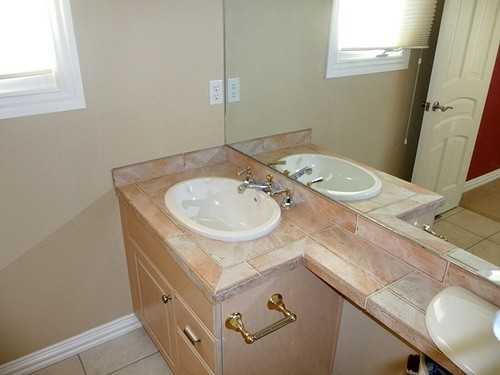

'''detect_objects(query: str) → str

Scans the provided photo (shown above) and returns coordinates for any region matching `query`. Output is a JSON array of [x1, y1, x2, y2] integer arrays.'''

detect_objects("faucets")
[[238, 174, 275, 196], [284, 166, 312, 181]]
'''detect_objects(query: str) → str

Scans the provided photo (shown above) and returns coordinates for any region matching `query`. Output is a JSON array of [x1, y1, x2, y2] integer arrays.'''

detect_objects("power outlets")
[[227, 78, 240, 102], [209, 80, 222, 106]]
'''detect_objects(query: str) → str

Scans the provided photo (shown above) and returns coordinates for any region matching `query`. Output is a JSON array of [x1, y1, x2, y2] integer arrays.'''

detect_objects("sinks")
[[276, 154, 382, 202], [164, 177, 281, 242]]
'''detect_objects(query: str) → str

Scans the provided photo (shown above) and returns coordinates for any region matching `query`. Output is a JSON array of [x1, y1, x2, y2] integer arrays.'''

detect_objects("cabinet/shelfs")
[[120, 202, 344, 375]]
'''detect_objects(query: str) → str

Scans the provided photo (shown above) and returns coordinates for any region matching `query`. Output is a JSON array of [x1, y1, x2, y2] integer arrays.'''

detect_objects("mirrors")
[[222, 0, 500, 285]]
